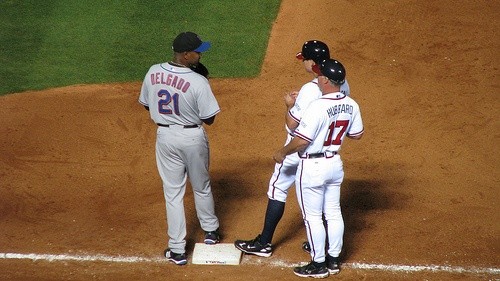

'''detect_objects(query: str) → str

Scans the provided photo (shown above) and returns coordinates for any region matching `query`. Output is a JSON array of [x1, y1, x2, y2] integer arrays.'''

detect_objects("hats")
[[172, 32, 211, 52]]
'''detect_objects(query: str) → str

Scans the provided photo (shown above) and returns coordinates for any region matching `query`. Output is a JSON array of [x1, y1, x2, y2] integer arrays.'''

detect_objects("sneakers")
[[302, 242, 326, 257], [293, 261, 328, 278], [325, 254, 339, 274], [204, 227, 221, 243], [234, 234, 272, 257], [164, 248, 187, 264]]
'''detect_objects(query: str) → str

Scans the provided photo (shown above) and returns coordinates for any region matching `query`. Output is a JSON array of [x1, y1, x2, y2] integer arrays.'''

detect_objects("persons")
[[139, 31, 223, 266], [235, 39, 350, 258], [273, 59, 364, 279]]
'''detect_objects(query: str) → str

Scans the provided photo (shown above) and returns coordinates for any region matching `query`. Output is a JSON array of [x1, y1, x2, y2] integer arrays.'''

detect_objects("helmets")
[[296, 40, 329, 64], [312, 59, 345, 84]]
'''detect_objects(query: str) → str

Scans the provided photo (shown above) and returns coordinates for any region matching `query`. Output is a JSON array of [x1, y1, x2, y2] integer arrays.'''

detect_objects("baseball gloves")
[[188, 62, 208, 77]]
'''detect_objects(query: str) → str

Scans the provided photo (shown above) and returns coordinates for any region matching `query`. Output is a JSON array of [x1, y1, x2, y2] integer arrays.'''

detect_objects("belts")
[[157, 123, 198, 128], [300, 152, 337, 158]]
